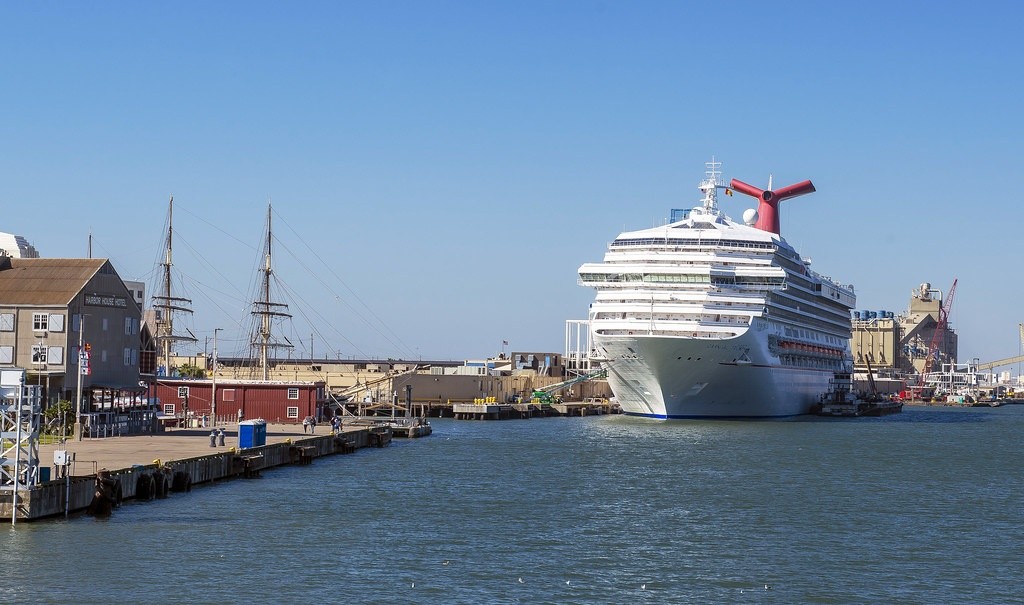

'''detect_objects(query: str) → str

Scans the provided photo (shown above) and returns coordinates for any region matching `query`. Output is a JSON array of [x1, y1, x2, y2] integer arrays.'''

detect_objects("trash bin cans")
[[238, 419, 266, 448]]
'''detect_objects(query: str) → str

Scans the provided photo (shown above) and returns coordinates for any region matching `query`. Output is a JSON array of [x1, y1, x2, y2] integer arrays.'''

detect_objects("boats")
[[810, 351, 904, 418]]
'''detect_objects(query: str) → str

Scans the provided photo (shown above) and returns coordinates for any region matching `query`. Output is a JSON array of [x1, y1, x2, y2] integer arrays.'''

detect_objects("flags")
[[725, 187, 733, 197], [503, 339, 508, 346], [79, 343, 92, 376]]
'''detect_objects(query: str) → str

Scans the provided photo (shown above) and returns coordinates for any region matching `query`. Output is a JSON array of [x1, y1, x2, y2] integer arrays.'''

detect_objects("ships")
[[578, 149, 856, 416]]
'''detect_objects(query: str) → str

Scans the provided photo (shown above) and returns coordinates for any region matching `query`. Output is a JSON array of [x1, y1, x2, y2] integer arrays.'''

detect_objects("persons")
[[302, 416, 308, 433], [201, 413, 207, 428], [330, 416, 343, 434], [308, 415, 317, 434]]
